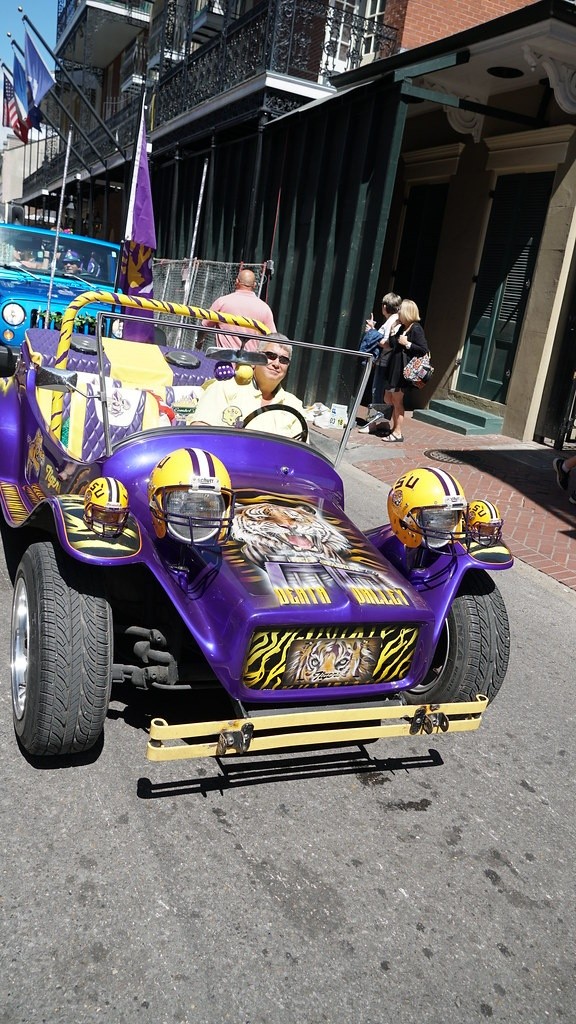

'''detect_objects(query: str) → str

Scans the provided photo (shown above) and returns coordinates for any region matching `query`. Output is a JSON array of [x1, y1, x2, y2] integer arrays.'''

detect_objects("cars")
[[0, 286, 513, 772]]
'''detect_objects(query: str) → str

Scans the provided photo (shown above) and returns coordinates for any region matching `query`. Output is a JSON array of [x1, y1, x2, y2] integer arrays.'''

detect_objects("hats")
[[61, 249, 83, 262]]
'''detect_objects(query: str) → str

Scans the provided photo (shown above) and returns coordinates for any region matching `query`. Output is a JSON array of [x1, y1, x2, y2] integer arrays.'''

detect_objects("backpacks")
[[400, 321, 436, 393]]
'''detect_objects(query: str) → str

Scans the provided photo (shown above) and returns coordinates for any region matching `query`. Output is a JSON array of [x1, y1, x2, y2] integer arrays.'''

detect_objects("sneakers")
[[552, 458, 572, 491], [569, 490, 576, 504], [357, 421, 377, 433], [376, 421, 391, 430]]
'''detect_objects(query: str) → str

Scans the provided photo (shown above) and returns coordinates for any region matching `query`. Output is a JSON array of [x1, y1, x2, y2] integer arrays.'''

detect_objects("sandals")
[[375, 430, 393, 436], [381, 433, 404, 442]]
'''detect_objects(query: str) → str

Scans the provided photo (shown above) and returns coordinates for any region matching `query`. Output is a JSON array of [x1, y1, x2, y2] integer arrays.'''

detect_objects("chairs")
[[40, 366, 169, 462], [157, 384, 204, 430]]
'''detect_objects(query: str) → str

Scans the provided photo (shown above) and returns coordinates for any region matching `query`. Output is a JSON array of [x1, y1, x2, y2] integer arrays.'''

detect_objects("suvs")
[[0, 223, 124, 374]]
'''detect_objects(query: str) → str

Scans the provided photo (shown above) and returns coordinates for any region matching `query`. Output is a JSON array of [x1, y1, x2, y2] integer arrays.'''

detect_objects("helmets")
[[146, 447, 234, 541], [387, 467, 469, 558], [83, 477, 129, 538], [467, 499, 502, 547]]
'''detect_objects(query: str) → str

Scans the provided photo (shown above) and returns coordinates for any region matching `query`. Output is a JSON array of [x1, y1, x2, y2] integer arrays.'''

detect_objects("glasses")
[[261, 350, 291, 364], [62, 261, 76, 265]]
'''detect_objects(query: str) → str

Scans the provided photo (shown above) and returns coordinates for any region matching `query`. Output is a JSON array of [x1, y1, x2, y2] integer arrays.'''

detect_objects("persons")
[[359, 292, 430, 442], [201, 269, 277, 354], [185, 331, 311, 453], [60, 253, 81, 274]]
